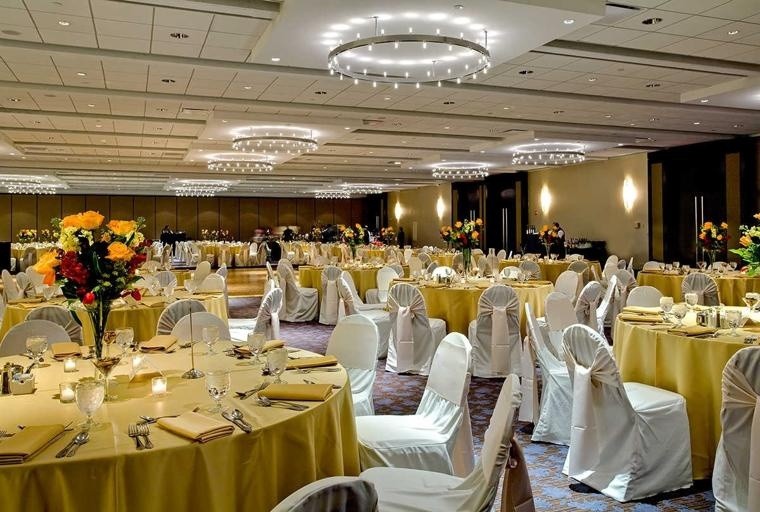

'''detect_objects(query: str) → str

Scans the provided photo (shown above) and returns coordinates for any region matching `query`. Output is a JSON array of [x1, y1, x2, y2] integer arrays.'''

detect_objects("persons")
[[282, 224, 294, 243], [550, 221, 566, 258], [361, 229, 370, 245], [396, 227, 405, 249], [161, 224, 173, 235], [320, 224, 337, 243]]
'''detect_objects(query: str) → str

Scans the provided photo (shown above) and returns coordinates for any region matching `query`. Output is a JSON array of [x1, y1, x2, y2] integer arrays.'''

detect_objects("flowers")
[[538, 224, 559, 250], [697, 213, 760, 276], [16, 228, 37, 243], [338, 223, 395, 250], [30, 210, 154, 357], [440, 218, 484, 274]]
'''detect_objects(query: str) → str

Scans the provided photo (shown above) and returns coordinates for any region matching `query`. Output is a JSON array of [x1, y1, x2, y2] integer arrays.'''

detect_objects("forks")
[[250, 394, 309, 412], [241, 382, 270, 400], [128, 424, 144, 451], [67, 434, 90, 458], [139, 423, 154, 450]]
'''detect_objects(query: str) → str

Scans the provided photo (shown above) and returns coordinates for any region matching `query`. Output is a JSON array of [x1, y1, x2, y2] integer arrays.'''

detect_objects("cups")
[[151, 377, 167, 397], [63, 357, 76, 372], [59, 382, 75, 402]]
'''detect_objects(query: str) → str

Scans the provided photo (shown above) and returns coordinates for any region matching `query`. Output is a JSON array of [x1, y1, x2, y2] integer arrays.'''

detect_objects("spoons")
[[304, 379, 341, 389], [140, 406, 201, 422], [232, 408, 252, 428], [236, 389, 257, 396]]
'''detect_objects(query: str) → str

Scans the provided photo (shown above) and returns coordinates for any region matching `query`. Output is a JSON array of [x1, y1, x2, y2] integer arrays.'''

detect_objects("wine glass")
[[410, 266, 536, 289], [660, 290, 760, 335], [184, 279, 197, 296], [25, 326, 294, 430], [44, 288, 53, 301], [279, 239, 482, 256], [658, 260, 739, 278], [312, 254, 401, 270], [163, 286, 174, 304], [508, 251, 587, 264]]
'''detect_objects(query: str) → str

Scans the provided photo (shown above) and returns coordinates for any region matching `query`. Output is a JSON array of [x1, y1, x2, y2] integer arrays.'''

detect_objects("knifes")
[[56, 429, 88, 459], [220, 412, 251, 434]]
